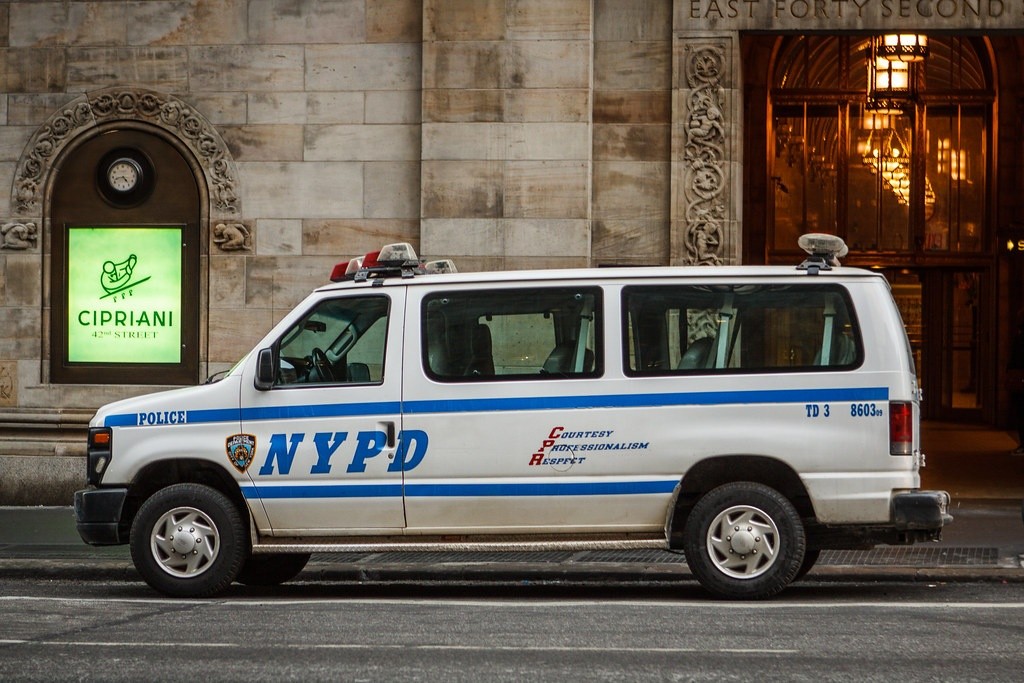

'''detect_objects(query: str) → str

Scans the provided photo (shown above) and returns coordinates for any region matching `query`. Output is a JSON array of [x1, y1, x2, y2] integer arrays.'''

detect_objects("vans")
[[72, 244, 956, 602]]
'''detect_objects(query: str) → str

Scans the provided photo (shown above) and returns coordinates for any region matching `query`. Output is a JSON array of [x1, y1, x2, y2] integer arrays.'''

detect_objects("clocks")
[[106, 158, 144, 194]]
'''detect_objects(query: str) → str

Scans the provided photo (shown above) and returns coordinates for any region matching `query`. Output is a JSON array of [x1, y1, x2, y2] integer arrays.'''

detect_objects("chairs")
[[679, 336, 714, 373], [537, 341, 596, 373], [425, 312, 453, 378], [464, 322, 496, 379], [813, 332, 856, 365]]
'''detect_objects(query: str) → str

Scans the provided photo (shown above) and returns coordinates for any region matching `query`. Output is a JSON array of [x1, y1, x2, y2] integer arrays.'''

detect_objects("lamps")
[[866, 57, 914, 117], [861, 112, 969, 207], [870, 46, 916, 98], [879, 32, 930, 63], [777, 124, 837, 189]]
